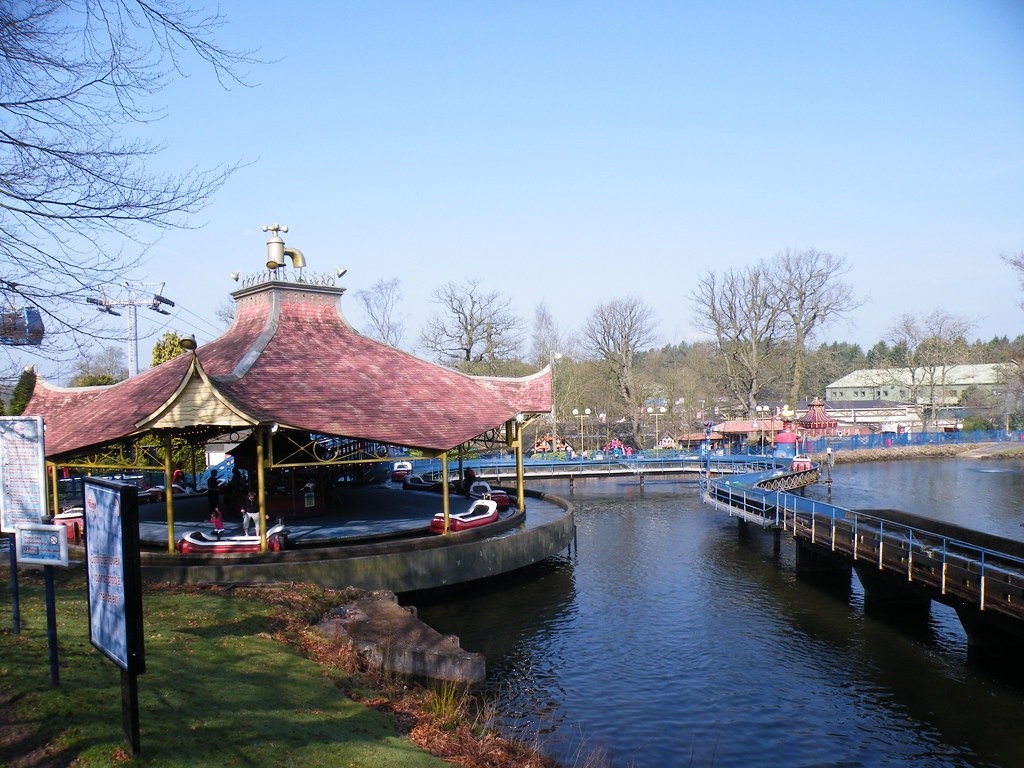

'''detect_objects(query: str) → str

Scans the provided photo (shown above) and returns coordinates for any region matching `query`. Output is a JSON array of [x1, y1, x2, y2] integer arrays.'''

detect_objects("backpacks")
[[464, 470, 475, 478]]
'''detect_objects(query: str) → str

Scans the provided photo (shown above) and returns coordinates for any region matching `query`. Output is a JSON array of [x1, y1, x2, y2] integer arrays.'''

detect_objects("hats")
[[211, 469, 218, 474]]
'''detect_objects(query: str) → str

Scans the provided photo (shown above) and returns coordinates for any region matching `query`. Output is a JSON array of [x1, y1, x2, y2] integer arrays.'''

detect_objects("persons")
[[208, 507, 225, 541], [241, 492, 260, 537], [208, 470, 223, 515], [222, 467, 241, 519], [463, 468, 476, 500]]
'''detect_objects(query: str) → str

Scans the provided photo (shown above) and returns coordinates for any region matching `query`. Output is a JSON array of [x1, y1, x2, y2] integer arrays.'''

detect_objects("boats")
[[392, 461, 413, 481], [469, 481, 509, 508], [428, 499, 499, 534], [138, 479, 227, 505], [402, 473, 455, 492], [53, 507, 84, 538], [176, 523, 287, 553]]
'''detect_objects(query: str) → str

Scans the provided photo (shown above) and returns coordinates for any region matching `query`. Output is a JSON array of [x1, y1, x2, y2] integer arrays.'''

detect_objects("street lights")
[[572, 406, 592, 463], [756, 405, 770, 455], [644, 406, 666, 457]]
